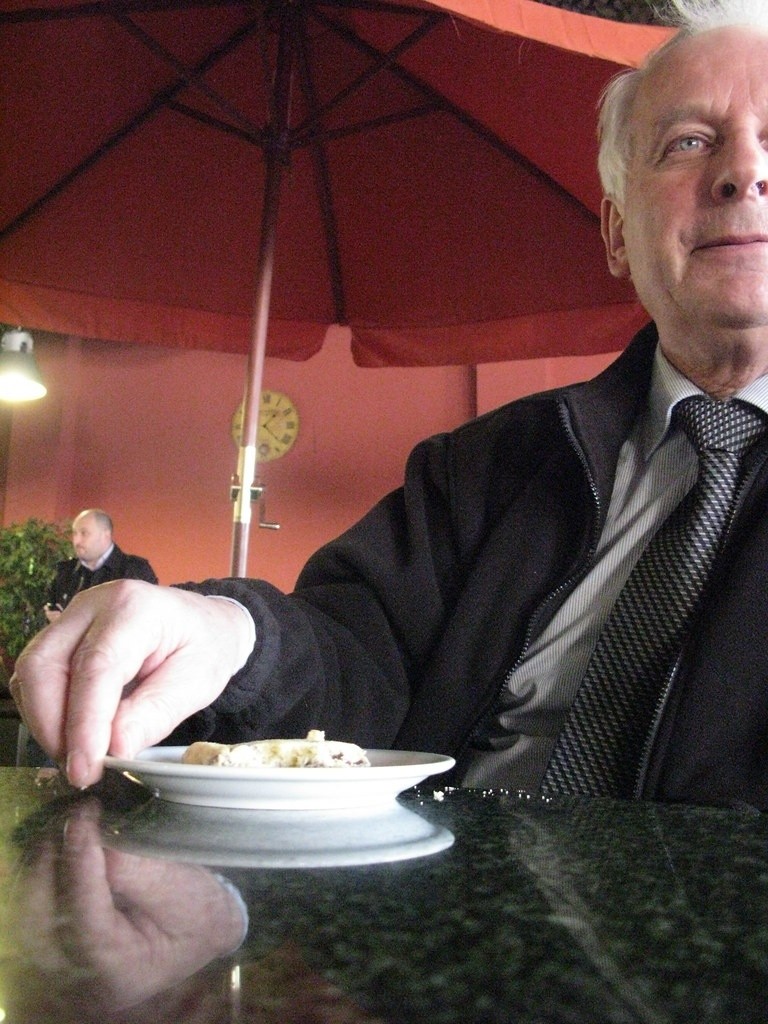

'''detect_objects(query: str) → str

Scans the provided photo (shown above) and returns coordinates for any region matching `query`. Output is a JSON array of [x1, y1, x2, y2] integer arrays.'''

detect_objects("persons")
[[10, 768, 768, 1024], [8, 23, 768, 797], [44, 509, 159, 622]]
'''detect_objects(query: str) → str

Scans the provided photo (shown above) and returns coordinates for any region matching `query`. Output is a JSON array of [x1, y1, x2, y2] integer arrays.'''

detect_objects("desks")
[[0, 763, 768, 1023]]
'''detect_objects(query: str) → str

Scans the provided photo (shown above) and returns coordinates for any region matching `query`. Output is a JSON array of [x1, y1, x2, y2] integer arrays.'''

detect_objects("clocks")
[[231, 387, 297, 462]]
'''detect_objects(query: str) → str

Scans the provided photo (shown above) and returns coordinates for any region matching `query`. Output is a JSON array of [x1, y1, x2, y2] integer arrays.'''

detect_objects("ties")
[[540, 400, 768, 800]]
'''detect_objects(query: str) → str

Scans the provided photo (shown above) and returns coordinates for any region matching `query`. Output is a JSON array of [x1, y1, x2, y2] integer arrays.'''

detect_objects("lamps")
[[0, 325, 46, 406]]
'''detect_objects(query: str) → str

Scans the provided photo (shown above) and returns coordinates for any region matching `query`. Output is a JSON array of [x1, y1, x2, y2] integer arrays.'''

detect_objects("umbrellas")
[[1, 1, 682, 590]]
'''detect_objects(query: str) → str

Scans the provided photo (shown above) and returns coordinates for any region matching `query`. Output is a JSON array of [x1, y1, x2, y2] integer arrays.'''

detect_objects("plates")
[[103, 746, 456, 809], [101, 798, 455, 870]]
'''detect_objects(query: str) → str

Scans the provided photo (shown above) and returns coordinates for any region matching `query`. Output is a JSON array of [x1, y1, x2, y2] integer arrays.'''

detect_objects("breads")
[[182, 730, 372, 769]]
